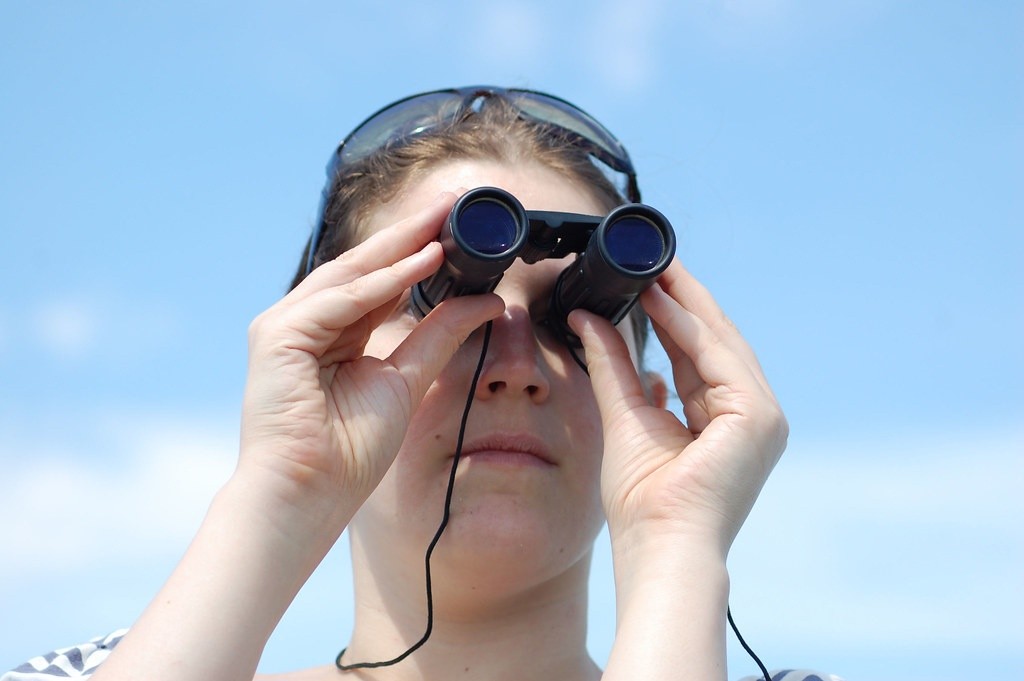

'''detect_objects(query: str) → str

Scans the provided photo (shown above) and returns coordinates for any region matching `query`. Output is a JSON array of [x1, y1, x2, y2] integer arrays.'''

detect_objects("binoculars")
[[410, 187, 675, 344]]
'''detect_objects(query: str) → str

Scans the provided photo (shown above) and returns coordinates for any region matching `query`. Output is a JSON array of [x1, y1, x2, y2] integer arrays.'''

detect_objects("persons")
[[0, 85, 828, 681]]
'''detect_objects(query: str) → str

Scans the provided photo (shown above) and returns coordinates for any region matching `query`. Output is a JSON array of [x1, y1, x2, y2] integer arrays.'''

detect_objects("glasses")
[[303, 85, 645, 285]]
